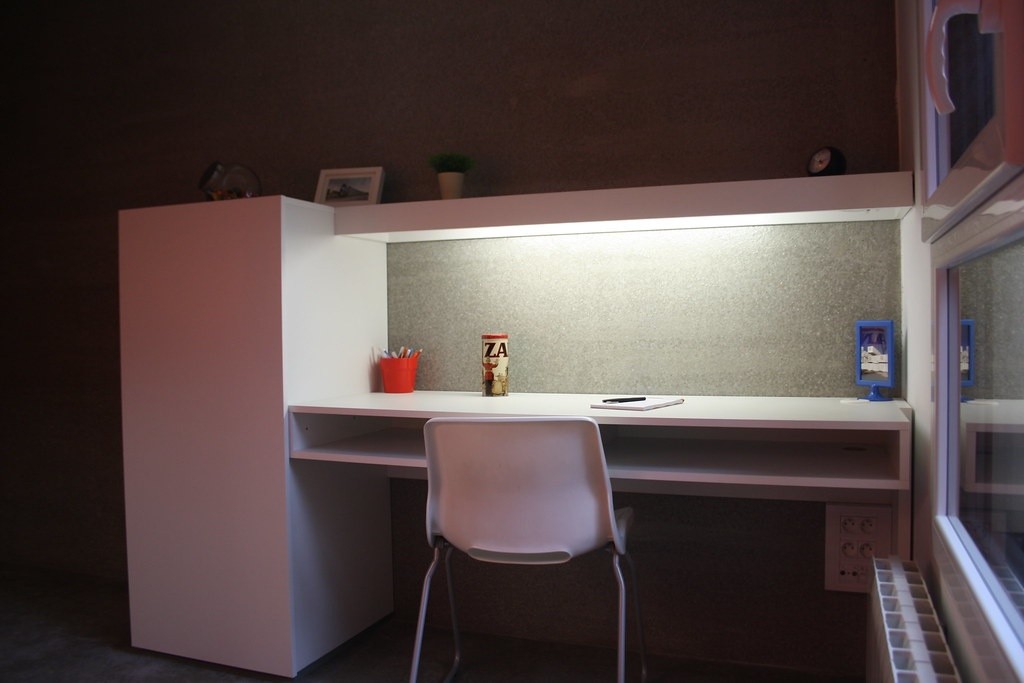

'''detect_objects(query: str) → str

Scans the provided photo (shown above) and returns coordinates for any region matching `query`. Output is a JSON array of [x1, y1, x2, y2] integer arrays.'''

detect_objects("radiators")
[[864, 556, 964, 683]]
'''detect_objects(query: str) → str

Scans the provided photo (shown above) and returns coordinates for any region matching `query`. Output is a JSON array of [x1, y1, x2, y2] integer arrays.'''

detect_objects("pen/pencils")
[[384, 346, 423, 358], [602, 397, 646, 403]]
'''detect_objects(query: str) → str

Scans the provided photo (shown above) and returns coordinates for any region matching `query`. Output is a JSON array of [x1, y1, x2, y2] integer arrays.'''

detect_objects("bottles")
[[199, 162, 262, 202], [482, 334, 509, 396]]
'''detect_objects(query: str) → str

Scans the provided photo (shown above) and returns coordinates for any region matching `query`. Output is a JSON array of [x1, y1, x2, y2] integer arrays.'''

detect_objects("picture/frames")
[[854, 321, 895, 387], [961, 319, 975, 388], [315, 165, 386, 206]]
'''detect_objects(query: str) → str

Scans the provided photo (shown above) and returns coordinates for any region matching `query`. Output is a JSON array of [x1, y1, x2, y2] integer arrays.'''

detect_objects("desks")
[[287, 392, 915, 560], [958, 400, 1024, 494]]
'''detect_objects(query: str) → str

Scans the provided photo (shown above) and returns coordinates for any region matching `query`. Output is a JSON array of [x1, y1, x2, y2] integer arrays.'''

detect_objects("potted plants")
[[431, 153, 468, 197]]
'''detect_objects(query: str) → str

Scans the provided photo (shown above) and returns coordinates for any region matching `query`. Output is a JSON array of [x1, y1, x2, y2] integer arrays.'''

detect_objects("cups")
[[380, 357, 418, 393]]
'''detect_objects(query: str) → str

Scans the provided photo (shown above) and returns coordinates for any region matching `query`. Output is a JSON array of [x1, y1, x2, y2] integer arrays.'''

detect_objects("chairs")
[[406, 412, 627, 682]]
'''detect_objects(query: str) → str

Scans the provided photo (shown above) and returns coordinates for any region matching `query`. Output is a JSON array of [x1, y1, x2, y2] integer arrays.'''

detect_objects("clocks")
[[805, 147, 845, 177]]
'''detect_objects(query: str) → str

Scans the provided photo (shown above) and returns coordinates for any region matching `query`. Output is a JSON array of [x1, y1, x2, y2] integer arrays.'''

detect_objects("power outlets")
[[824, 502, 892, 593]]
